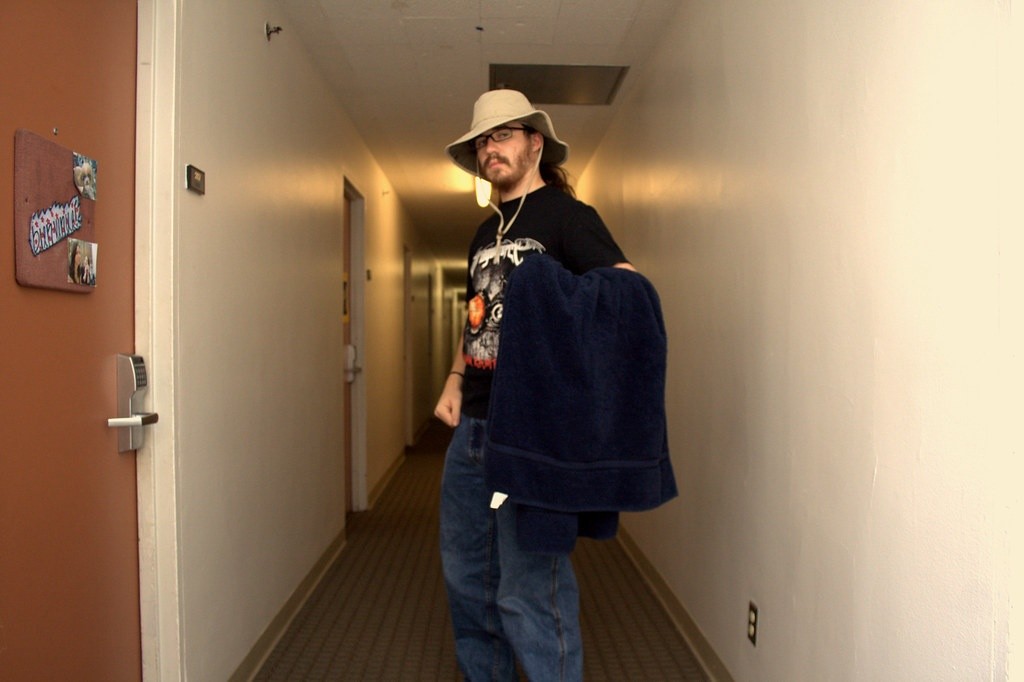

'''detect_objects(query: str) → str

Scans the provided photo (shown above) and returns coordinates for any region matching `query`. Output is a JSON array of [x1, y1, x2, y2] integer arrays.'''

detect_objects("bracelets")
[[450, 370, 465, 378]]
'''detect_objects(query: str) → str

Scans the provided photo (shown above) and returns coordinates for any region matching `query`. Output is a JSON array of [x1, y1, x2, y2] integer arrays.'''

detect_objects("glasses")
[[475, 125, 527, 149]]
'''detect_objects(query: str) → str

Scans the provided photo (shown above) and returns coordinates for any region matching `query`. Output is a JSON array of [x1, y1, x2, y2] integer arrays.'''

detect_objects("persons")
[[434, 88, 637, 682]]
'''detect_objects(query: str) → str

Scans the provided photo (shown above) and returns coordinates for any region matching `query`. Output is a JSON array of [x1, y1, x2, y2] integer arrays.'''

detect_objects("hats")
[[443, 89, 569, 182]]
